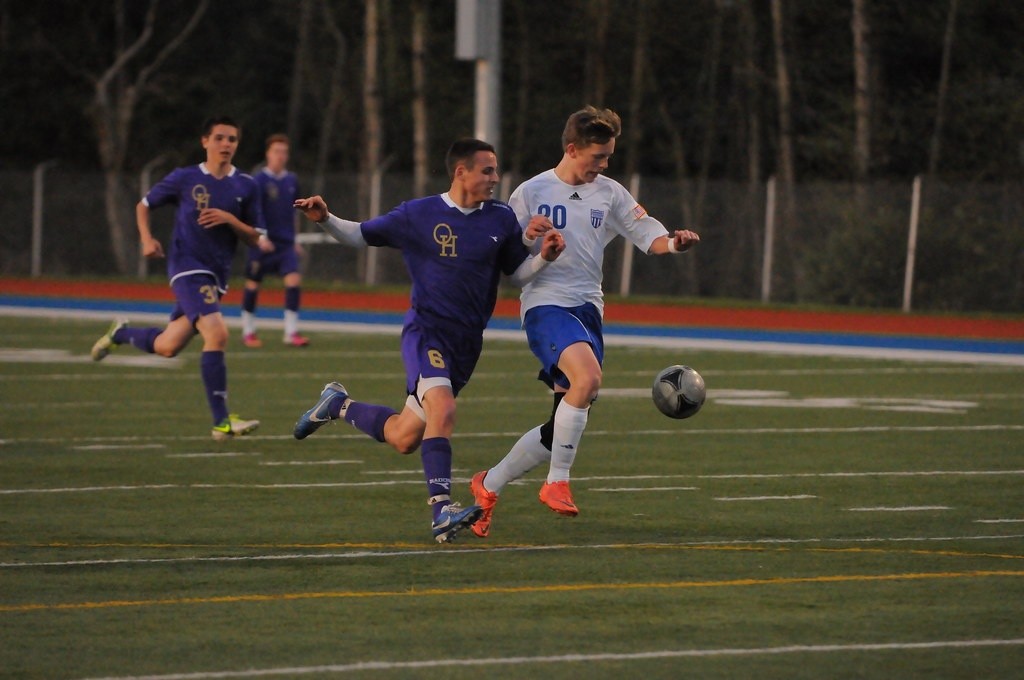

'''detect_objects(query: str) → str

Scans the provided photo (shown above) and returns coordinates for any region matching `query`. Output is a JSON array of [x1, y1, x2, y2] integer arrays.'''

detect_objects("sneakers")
[[539, 480, 579, 518], [92, 315, 129, 363], [244, 333, 262, 347], [282, 333, 310, 346], [431, 502, 483, 543], [293, 381, 348, 440], [469, 471, 498, 538], [212, 412, 260, 443]]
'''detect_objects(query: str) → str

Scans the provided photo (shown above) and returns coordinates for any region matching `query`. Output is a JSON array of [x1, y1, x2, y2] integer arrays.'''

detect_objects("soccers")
[[652, 363, 708, 420]]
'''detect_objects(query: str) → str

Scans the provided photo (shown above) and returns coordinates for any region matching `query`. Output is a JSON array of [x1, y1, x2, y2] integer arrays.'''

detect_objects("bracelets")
[[522, 226, 537, 246], [668, 238, 688, 255]]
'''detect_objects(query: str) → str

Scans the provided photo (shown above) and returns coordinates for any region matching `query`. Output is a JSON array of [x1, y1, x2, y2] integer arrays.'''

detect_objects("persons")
[[469, 105, 700, 537], [240, 134, 313, 349], [294, 136, 567, 545], [90, 114, 266, 439]]
